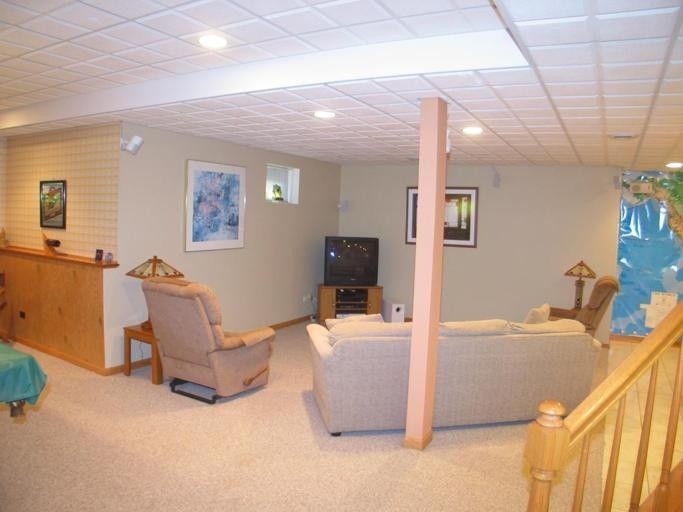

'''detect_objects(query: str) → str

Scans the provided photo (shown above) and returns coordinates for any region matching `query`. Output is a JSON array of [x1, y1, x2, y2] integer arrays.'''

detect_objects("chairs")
[[573, 274, 619, 337], [142, 277, 277, 407]]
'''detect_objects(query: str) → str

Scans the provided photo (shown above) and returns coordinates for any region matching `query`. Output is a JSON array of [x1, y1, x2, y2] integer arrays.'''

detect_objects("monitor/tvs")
[[324, 236, 380, 286]]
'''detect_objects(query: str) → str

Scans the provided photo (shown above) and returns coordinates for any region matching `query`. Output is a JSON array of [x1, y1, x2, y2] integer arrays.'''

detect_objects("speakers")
[[383, 297, 405, 323]]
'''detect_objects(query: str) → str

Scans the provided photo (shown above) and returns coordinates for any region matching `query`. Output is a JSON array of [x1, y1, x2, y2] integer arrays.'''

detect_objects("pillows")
[[525, 302, 550, 323], [323, 313, 384, 331]]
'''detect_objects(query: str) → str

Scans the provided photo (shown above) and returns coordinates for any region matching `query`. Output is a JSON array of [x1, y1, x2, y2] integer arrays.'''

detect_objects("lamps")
[[562, 261, 596, 310], [126, 255, 184, 331], [119, 132, 146, 157]]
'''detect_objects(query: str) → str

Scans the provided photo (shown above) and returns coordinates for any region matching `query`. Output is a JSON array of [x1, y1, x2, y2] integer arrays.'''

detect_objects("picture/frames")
[[183, 158, 247, 253], [405, 185, 478, 248], [39, 180, 67, 231]]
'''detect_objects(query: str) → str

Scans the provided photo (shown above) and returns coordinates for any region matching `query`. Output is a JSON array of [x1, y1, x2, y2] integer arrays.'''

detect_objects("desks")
[[123, 320, 165, 384], [549, 307, 577, 319], [0, 341, 50, 419]]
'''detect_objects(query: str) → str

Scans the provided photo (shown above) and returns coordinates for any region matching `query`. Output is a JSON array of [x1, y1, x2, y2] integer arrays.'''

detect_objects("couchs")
[[306, 319, 599, 438]]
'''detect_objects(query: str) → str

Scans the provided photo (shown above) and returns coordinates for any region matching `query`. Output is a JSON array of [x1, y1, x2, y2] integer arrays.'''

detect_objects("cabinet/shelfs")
[[317, 284, 384, 324]]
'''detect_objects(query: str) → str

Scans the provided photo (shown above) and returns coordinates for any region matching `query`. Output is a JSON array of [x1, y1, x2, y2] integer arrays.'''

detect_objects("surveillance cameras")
[[125, 134, 146, 156]]
